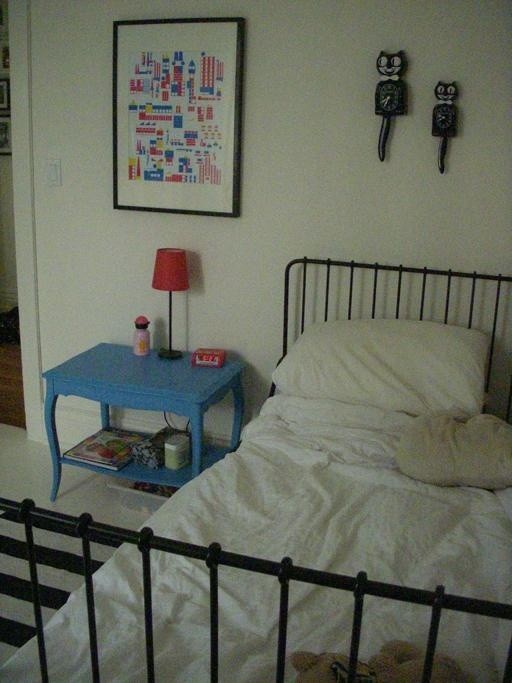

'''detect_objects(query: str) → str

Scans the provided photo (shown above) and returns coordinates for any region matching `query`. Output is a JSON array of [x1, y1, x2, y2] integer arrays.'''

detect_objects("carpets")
[[0, 508, 124, 663]]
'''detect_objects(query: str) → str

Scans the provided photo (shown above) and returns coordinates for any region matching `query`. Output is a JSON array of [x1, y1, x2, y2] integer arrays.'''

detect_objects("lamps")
[[151, 246, 190, 360]]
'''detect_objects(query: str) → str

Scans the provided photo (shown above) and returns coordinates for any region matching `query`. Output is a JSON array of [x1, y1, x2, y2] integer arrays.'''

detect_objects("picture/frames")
[[112, 15, 247, 219]]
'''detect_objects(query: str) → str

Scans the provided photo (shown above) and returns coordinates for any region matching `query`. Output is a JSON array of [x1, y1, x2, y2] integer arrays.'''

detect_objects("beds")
[[0, 255, 512, 682]]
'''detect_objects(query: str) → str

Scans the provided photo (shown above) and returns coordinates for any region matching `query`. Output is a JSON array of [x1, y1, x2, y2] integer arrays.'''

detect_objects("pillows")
[[268, 318, 492, 419]]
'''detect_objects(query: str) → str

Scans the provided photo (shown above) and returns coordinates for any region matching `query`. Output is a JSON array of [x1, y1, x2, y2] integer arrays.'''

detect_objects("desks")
[[38, 341, 247, 503]]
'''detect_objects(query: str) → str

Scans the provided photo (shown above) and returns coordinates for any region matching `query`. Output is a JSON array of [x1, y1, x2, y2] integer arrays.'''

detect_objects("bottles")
[[132, 314, 153, 357]]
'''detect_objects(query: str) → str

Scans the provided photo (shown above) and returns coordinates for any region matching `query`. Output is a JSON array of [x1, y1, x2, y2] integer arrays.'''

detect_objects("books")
[[63, 424, 151, 472]]
